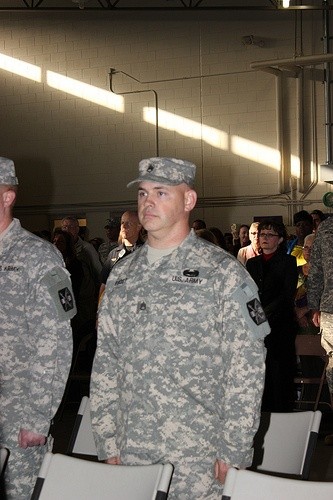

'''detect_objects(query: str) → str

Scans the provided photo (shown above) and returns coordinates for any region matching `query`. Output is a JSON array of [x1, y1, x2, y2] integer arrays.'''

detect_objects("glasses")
[[259, 232, 280, 237]]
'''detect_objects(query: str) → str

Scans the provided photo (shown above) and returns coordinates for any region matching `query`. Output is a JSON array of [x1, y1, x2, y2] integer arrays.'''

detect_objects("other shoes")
[[324, 434, 333, 444]]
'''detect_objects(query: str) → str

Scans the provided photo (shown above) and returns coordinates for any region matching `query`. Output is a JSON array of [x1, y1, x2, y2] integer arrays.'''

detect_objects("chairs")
[[28, 332, 332, 499]]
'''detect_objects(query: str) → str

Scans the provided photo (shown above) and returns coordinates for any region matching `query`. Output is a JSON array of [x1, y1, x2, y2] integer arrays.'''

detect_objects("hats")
[[126, 156, 197, 188], [0, 157, 19, 186], [103, 216, 120, 227]]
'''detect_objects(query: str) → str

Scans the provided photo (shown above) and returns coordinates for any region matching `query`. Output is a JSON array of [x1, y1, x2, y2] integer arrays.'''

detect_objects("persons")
[[50, 210, 333, 414], [89, 157, 272, 500], [0, 156, 78, 500]]
